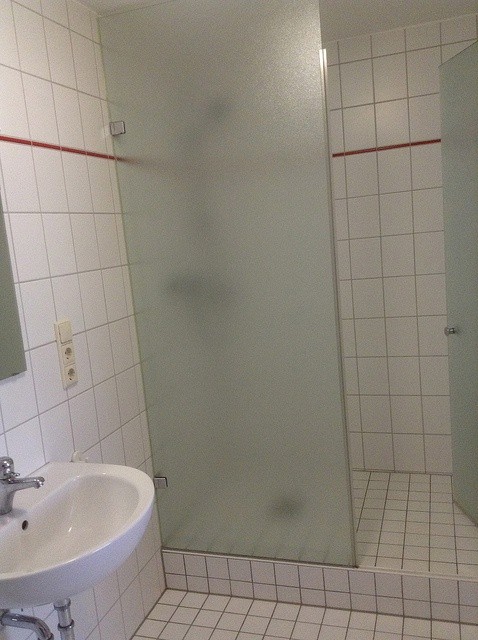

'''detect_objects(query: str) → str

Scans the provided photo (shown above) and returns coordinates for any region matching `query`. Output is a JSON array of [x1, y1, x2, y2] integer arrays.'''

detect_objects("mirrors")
[[0, 194, 27, 379]]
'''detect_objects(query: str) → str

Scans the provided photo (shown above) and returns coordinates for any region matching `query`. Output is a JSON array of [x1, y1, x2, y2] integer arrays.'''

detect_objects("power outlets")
[[58, 342, 78, 389]]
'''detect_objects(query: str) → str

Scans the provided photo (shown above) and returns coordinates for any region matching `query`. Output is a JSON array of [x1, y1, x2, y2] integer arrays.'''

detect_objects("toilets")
[[0, 462, 156, 613]]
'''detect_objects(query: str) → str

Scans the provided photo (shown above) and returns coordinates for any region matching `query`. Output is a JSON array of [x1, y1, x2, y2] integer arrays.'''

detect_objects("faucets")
[[0, 457, 45, 515]]
